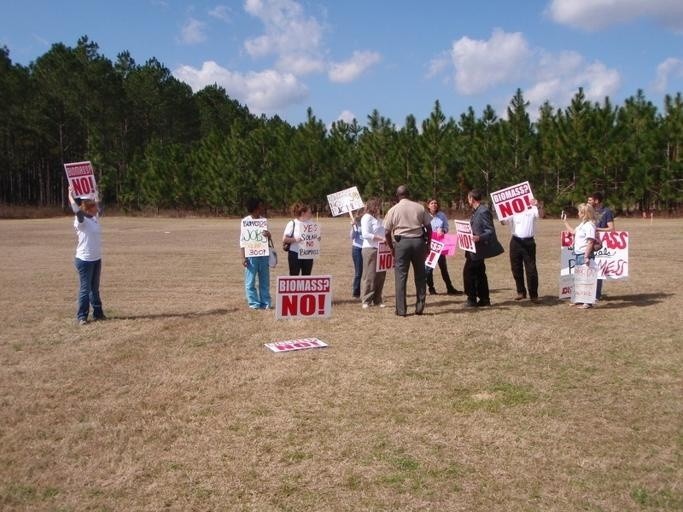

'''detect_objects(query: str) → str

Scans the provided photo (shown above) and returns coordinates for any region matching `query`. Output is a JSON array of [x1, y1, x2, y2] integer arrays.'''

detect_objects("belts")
[[513, 237, 534, 240]]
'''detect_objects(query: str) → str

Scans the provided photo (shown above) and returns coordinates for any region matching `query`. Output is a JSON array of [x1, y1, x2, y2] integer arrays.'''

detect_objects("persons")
[[282, 201, 321, 276], [348, 207, 363, 299], [239, 197, 272, 310], [382, 186, 432, 317], [423, 198, 463, 296], [463, 190, 505, 309], [561, 203, 602, 310], [587, 193, 615, 302], [501, 199, 544, 305], [361, 198, 387, 309], [68, 185, 111, 325]]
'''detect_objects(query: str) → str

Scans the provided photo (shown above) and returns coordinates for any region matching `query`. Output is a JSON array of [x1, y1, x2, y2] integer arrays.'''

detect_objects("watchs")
[[584, 256, 589, 259], [538, 205, 543, 209]]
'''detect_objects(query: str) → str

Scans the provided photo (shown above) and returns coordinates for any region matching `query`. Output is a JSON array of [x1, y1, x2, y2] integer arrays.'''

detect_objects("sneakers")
[[578, 304, 591, 309], [569, 302, 581, 307], [374, 302, 386, 308], [362, 303, 369, 308]]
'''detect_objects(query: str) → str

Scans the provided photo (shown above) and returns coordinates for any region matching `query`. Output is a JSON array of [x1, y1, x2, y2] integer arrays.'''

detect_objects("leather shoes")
[[461, 300, 477, 307], [395, 310, 404, 316], [477, 299, 490, 306], [531, 296, 540, 303], [415, 310, 422, 315], [429, 287, 436, 294], [448, 288, 463, 295], [514, 294, 526, 300]]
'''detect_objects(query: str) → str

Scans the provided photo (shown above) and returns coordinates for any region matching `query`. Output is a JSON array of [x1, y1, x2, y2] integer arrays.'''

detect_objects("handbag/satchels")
[[282, 219, 295, 251], [269, 239, 277, 268]]
